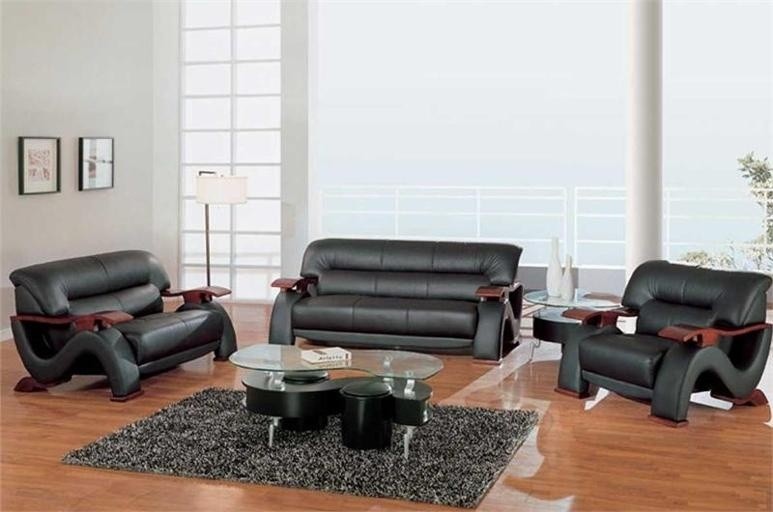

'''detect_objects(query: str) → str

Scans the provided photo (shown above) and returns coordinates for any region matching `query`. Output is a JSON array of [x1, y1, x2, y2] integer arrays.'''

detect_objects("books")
[[300, 346, 352, 364]]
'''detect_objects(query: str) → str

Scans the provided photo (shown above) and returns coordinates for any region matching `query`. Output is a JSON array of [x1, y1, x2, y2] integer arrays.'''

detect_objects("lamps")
[[192, 169, 249, 329]]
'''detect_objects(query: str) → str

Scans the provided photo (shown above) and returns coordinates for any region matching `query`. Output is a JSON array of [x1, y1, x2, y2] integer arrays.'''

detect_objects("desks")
[[522, 288, 624, 359], [227, 340, 447, 452]]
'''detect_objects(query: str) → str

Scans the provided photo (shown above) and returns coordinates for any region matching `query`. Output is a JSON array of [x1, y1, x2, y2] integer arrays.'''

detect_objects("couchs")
[[555, 257, 770, 427], [266, 238, 527, 366], [9, 249, 238, 403]]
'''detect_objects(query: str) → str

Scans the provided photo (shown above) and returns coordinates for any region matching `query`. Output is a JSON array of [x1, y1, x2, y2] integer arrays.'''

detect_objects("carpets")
[[63, 383, 542, 508]]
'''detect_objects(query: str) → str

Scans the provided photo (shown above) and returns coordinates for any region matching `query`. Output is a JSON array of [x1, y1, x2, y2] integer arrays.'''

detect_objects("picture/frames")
[[17, 136, 64, 195], [77, 137, 115, 193]]
